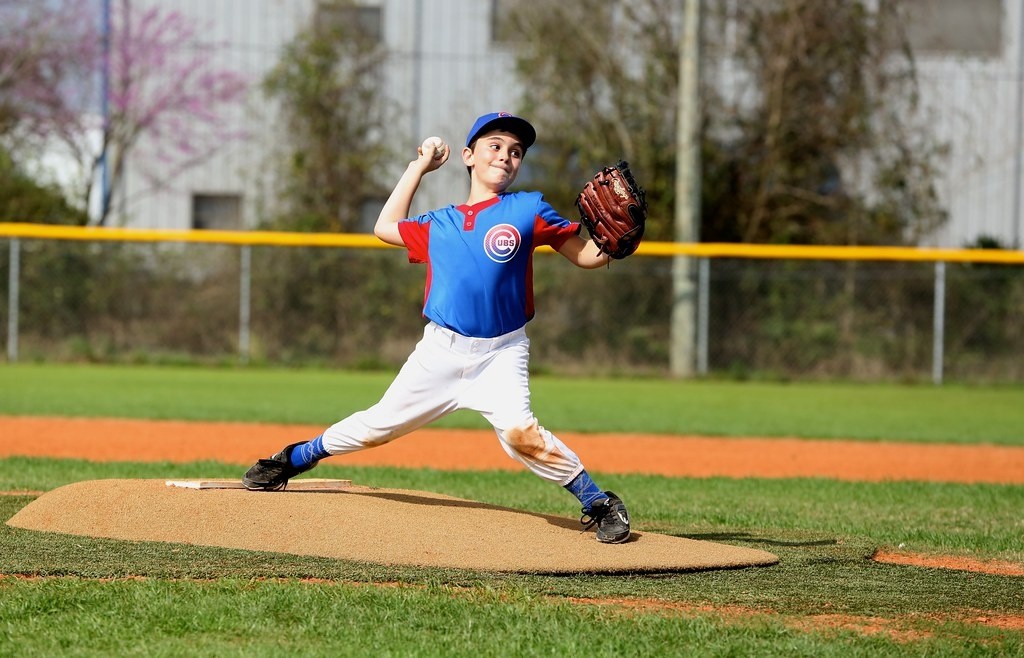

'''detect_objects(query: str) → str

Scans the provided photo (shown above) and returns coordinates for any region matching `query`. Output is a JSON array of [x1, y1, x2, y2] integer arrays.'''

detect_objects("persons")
[[240, 111, 648, 544]]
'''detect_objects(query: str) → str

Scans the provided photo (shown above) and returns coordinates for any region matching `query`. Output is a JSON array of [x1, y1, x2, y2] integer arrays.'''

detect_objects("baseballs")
[[422, 137, 444, 158]]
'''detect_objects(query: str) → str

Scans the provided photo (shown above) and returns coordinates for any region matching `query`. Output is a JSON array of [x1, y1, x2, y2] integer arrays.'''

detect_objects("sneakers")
[[580, 491, 630, 544], [241, 441, 318, 491]]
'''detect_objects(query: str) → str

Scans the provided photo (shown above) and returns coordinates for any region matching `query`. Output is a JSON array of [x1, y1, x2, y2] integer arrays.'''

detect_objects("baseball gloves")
[[577, 160, 646, 260]]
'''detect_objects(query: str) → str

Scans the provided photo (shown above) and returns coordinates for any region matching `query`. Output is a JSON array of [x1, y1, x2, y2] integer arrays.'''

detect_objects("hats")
[[466, 113, 537, 151]]
